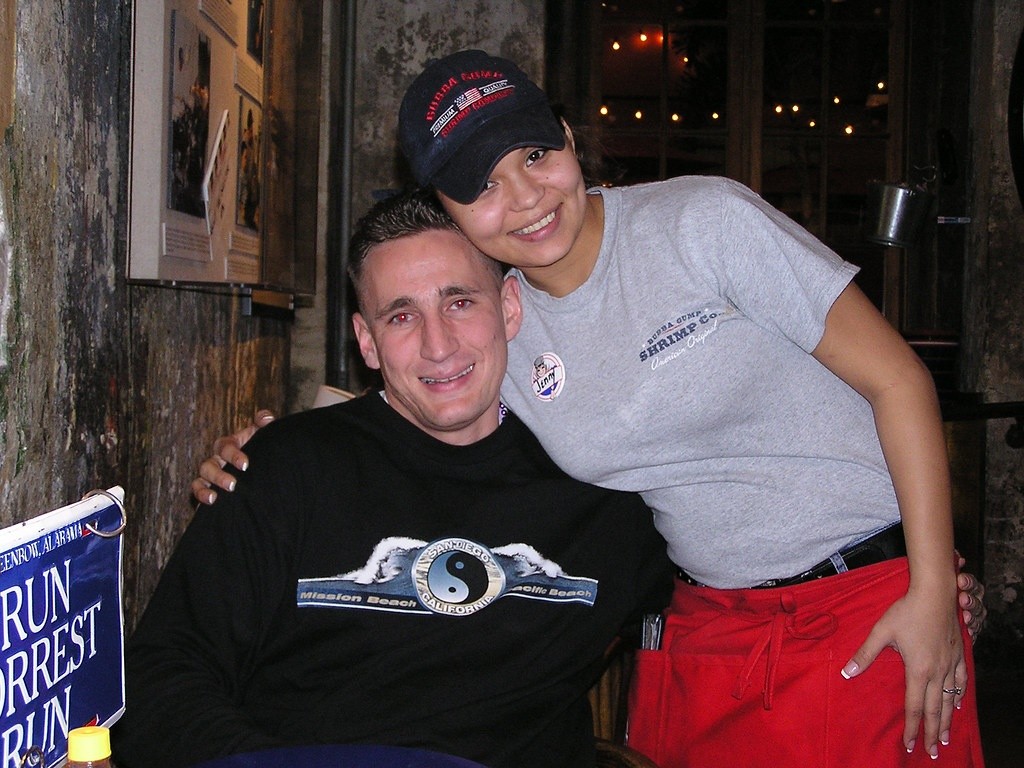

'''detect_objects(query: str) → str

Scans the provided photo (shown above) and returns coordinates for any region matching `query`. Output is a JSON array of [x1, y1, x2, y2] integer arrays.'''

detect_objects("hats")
[[398, 49, 566, 205]]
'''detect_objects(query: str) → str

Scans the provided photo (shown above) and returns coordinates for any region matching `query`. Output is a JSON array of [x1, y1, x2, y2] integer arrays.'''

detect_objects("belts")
[[677, 521, 907, 590]]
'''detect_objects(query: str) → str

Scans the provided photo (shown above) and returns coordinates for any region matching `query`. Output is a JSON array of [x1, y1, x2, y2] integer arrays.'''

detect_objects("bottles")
[[63, 725, 112, 768]]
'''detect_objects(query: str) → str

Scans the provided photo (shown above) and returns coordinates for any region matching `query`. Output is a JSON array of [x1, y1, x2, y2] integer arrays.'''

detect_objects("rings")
[[942, 687, 962, 695]]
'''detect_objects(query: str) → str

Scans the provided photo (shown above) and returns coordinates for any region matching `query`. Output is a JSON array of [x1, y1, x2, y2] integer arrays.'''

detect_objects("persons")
[[193, 51, 983, 768], [110, 186, 987, 768]]
